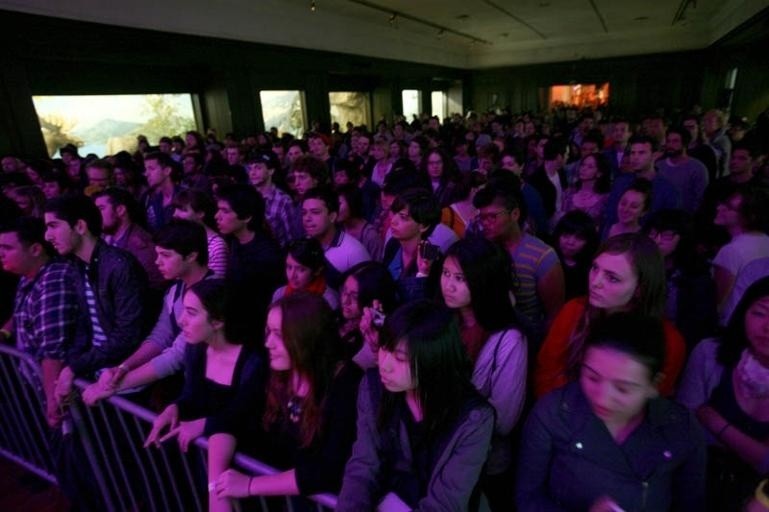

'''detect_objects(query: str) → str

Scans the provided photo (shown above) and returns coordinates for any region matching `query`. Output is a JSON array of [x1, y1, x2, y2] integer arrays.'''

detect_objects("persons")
[[475, 134, 491, 152], [549, 208, 596, 295], [427, 116, 441, 132], [258, 133, 271, 152], [579, 132, 602, 157], [42, 173, 72, 202], [435, 236, 530, 445], [525, 121, 537, 139], [653, 126, 709, 212], [179, 154, 208, 197], [703, 108, 733, 178], [467, 143, 514, 190], [380, 140, 404, 184], [206, 289, 363, 512], [454, 139, 478, 175], [610, 152, 635, 177], [158, 137, 171, 153], [511, 311, 695, 511], [0, 215, 86, 424], [501, 149, 545, 237], [63, 157, 112, 217], [67, 158, 81, 178], [223, 133, 236, 149], [303, 120, 321, 139], [293, 156, 328, 197], [393, 123, 406, 141], [202, 144, 223, 173], [525, 137, 537, 160], [270, 144, 285, 166], [246, 135, 256, 151], [333, 159, 378, 223], [247, 150, 298, 248], [704, 146, 761, 242], [1, 155, 17, 173], [382, 187, 443, 303], [351, 125, 369, 137], [136, 135, 148, 155], [425, 128, 442, 148], [170, 188, 232, 280], [561, 114, 594, 157], [603, 135, 680, 243], [287, 143, 305, 167], [338, 136, 375, 179], [108, 159, 137, 193], [42, 194, 154, 509], [527, 139, 570, 239], [525, 135, 549, 176], [596, 177, 653, 257], [13, 186, 45, 219], [373, 120, 393, 141], [561, 152, 609, 223], [680, 115, 719, 180], [541, 122, 551, 138], [331, 122, 343, 139], [419, 147, 458, 207], [270, 126, 280, 143], [143, 279, 258, 454], [372, 140, 392, 185], [60, 143, 79, 166], [334, 299, 510, 512], [641, 208, 720, 338], [169, 136, 185, 154], [185, 131, 204, 155], [272, 238, 339, 314], [335, 183, 378, 258], [213, 184, 283, 285], [0, 171, 33, 200], [492, 120, 506, 138], [464, 129, 474, 147], [509, 119, 524, 139], [206, 128, 216, 144], [440, 170, 488, 238], [384, 136, 429, 192], [139, 151, 184, 232], [302, 186, 371, 279], [343, 122, 354, 143], [678, 276, 769, 479], [337, 258, 394, 370], [709, 187, 769, 331], [687, 104, 703, 120], [81, 219, 217, 408], [471, 180, 565, 327], [225, 144, 245, 170], [307, 133, 334, 171], [604, 120, 633, 172], [637, 116, 656, 137], [90, 186, 162, 281]]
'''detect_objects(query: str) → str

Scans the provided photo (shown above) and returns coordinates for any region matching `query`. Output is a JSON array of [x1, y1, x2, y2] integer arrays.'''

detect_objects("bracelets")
[[247, 476, 253, 497], [208, 481, 217, 493], [717, 423, 731, 438]]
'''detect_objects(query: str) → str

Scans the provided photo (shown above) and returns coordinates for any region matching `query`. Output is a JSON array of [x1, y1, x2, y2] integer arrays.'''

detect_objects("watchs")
[[118, 364, 127, 372]]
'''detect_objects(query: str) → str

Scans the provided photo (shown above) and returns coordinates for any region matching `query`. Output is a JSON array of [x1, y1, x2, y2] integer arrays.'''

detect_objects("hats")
[[59, 143, 79, 157]]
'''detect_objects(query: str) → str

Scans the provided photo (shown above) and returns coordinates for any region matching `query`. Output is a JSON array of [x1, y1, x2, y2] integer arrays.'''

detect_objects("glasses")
[[182, 159, 194, 164], [479, 207, 509, 222]]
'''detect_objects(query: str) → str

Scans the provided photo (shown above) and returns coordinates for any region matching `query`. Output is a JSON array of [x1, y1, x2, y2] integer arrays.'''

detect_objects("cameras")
[[419, 243, 440, 261], [371, 310, 388, 326]]
